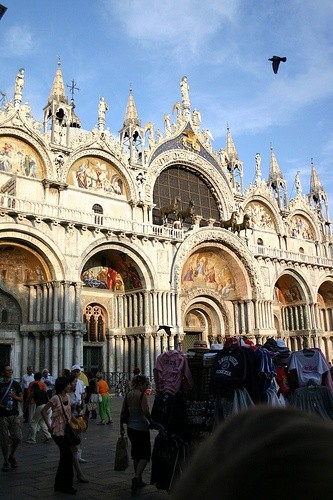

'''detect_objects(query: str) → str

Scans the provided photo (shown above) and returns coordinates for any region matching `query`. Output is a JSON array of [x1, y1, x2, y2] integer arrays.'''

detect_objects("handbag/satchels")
[[86, 403, 98, 412], [120, 408, 130, 424], [66, 424, 81, 447], [114, 436, 129, 472], [67, 416, 87, 433]]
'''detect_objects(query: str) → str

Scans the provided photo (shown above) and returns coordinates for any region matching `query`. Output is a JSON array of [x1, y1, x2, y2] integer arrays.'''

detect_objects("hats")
[[71, 365, 80, 371]]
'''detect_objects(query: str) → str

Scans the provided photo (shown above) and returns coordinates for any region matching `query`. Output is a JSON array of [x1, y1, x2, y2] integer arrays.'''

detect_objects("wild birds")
[[268, 55, 287, 75]]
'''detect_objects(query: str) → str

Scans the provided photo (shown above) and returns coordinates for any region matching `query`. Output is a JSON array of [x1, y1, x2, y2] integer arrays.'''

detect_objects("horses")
[[159, 194, 182, 221], [177, 199, 196, 225], [231, 213, 254, 238], [221, 211, 240, 232]]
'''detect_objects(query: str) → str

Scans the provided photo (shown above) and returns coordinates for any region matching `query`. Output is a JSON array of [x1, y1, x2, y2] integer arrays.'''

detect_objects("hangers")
[[292, 340, 317, 357], [224, 337, 275, 352]]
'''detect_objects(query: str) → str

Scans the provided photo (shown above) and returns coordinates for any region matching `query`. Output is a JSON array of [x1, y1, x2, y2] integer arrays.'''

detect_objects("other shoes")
[[8, 455, 18, 468], [98, 419, 106, 425], [78, 456, 87, 463], [77, 473, 88, 484], [2, 462, 9, 472], [54, 485, 78, 493], [26, 439, 36, 444], [108, 419, 114, 423], [131, 477, 145, 490]]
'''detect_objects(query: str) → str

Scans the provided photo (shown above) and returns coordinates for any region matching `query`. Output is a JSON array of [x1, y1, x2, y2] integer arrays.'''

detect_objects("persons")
[[169, 404, 333, 500], [0, 365, 155, 493], [14, 68, 302, 194]]
